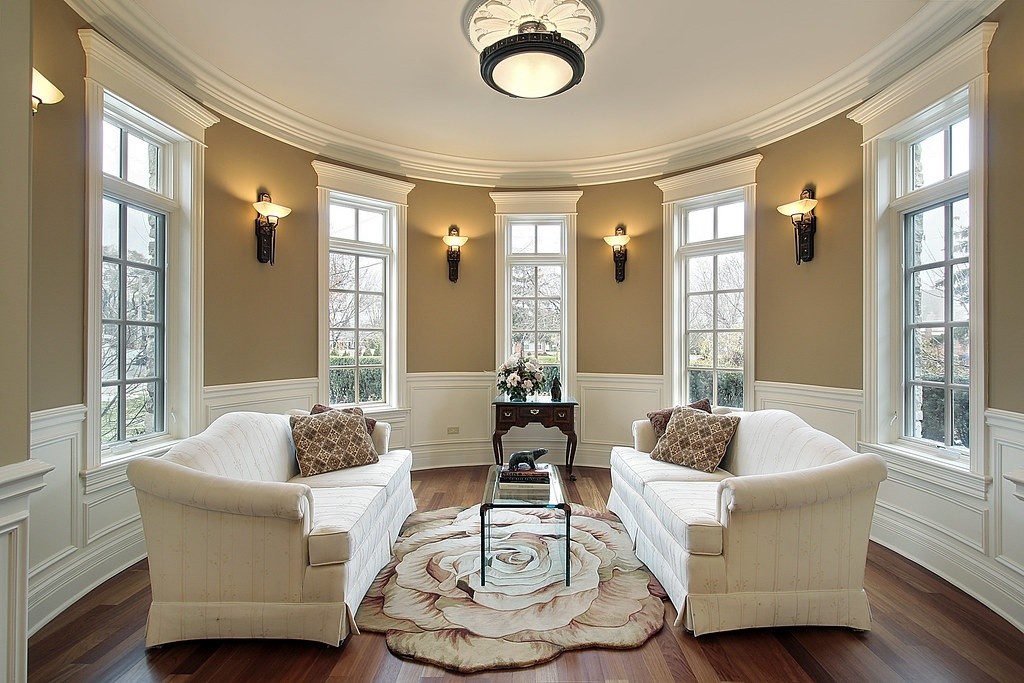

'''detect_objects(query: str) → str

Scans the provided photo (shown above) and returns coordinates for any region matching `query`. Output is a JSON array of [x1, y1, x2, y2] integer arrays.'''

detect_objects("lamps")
[[461, 0, 603, 100], [442, 225, 468, 284], [777, 188, 819, 266], [252, 192, 291, 267], [32, 66, 65, 116], [603, 224, 631, 283]]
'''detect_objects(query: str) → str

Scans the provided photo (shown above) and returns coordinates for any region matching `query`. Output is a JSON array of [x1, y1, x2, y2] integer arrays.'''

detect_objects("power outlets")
[[447, 427, 459, 434]]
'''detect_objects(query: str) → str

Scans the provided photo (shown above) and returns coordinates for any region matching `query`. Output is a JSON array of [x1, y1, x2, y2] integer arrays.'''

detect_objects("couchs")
[[126, 409, 416, 648], [606, 407, 888, 637]]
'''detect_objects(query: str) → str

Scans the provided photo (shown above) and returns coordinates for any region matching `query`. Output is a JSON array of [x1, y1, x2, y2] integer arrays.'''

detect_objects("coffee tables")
[[480, 463, 572, 587]]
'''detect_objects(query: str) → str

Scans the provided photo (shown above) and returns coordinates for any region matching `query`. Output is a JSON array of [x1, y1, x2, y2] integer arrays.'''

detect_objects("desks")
[[492, 396, 579, 481]]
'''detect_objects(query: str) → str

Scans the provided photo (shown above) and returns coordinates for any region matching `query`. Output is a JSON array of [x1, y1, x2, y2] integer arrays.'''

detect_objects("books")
[[498, 463, 551, 491]]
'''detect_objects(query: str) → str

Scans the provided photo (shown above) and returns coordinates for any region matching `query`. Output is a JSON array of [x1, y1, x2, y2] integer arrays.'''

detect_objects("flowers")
[[497, 353, 546, 402]]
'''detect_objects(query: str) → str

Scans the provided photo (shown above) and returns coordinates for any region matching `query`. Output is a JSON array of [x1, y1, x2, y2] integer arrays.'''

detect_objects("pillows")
[[309, 403, 377, 436], [650, 404, 741, 474], [647, 398, 712, 443], [290, 407, 379, 477]]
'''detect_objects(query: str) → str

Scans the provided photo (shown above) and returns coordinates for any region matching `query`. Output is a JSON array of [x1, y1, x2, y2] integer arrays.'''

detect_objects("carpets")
[[355, 499, 670, 672]]
